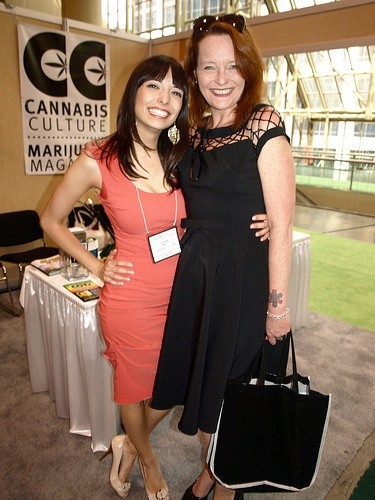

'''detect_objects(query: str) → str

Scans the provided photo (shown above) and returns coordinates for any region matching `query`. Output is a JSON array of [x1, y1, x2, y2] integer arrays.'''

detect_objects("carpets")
[[0, 228, 375, 500]]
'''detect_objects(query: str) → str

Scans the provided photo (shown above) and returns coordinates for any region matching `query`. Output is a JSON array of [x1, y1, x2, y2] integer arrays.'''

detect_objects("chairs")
[[0, 204, 115, 317]]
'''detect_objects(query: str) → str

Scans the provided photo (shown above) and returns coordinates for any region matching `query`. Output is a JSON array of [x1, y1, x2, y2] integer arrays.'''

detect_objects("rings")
[[276, 337, 283, 341]]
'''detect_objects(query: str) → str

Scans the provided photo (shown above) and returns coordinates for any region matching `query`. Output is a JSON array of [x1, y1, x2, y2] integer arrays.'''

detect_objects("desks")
[[19, 229, 310, 453]]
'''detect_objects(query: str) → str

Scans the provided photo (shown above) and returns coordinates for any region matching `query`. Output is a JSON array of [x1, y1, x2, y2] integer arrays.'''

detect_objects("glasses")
[[194, 13, 246, 34], [188, 127, 206, 182]]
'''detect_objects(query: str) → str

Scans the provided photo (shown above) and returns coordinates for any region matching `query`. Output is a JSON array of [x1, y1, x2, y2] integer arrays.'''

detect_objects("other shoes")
[[181, 473, 245, 500]]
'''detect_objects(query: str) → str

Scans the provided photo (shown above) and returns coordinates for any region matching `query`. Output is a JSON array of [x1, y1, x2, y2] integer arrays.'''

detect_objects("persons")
[[40, 55, 271, 500], [148, 13, 297, 500]]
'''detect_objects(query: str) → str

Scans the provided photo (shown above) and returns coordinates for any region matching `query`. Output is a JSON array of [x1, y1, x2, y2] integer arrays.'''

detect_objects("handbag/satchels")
[[205, 331, 332, 492]]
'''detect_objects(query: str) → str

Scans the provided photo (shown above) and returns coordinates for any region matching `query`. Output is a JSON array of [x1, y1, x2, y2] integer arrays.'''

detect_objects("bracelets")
[[266, 307, 291, 320]]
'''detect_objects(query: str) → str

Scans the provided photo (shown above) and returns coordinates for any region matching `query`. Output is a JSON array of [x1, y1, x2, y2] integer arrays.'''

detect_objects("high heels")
[[98, 434, 132, 500], [138, 455, 170, 500]]
